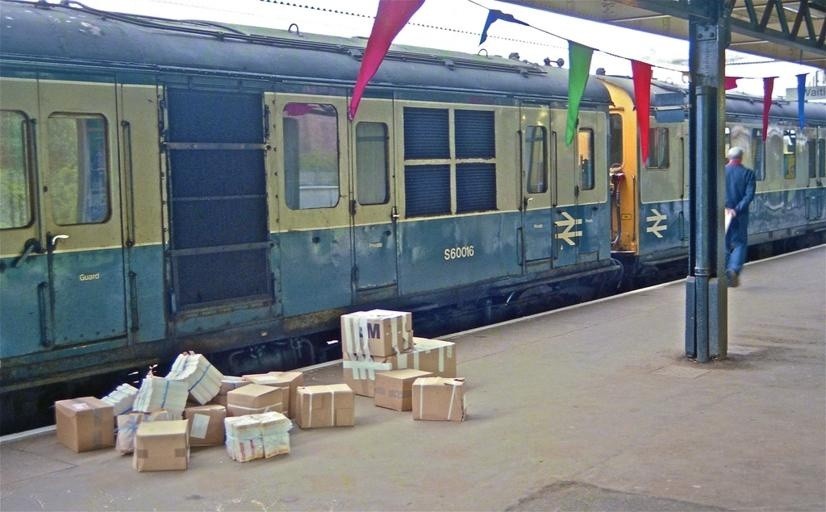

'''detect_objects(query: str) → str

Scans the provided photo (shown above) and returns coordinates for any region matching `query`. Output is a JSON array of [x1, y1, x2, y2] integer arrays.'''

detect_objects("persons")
[[723, 146, 757, 286]]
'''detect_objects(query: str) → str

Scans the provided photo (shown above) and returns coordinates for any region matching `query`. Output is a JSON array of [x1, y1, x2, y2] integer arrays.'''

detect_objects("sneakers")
[[726, 268, 738, 287]]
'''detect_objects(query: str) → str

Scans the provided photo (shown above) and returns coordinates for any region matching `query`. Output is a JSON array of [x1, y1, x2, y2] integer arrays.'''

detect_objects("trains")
[[1, 0, 826, 438]]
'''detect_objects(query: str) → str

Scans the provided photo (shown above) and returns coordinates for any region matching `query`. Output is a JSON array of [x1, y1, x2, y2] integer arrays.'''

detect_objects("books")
[[725, 208, 733, 235]]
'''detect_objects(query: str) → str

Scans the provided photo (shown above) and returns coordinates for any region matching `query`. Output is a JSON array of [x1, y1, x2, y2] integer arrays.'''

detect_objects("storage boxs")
[[370, 367, 434, 414], [411, 375, 465, 425], [339, 310, 460, 399], [60, 348, 307, 469], [295, 382, 355, 430]]
